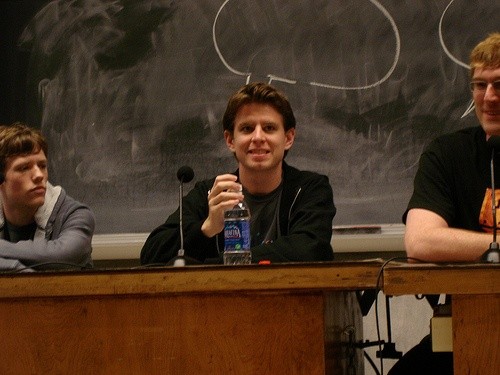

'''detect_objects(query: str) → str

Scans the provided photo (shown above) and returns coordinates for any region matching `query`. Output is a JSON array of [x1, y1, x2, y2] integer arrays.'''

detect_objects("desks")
[[0, 256, 384, 375], [385, 259, 500, 375]]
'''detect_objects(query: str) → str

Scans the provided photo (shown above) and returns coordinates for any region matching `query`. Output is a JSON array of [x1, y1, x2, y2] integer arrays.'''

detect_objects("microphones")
[[487, 135, 500, 249], [177, 165, 194, 256]]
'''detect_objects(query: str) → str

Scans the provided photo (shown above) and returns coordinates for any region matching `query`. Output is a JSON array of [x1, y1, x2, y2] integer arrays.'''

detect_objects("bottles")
[[223, 184, 252, 265]]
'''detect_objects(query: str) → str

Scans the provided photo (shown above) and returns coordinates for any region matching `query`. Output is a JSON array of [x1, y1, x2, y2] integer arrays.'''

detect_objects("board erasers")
[[333, 226, 382, 236]]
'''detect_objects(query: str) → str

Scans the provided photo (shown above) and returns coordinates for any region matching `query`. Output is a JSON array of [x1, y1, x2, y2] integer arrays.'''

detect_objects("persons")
[[399, 30, 500, 262], [140, 82, 337, 263], [0, 121, 95, 275]]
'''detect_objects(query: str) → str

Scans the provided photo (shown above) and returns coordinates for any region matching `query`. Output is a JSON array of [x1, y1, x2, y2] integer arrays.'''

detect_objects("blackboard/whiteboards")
[[0, 1, 499, 258]]
